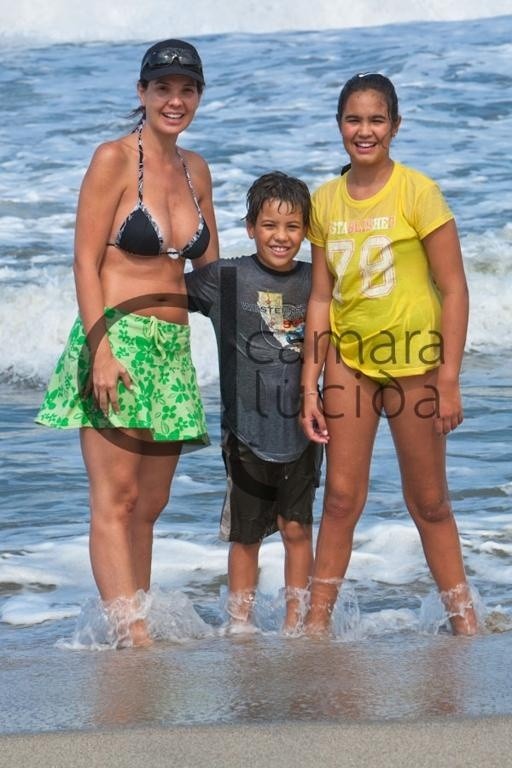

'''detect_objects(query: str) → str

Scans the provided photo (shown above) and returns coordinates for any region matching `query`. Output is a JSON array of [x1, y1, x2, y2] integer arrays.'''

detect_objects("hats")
[[139, 39, 204, 84]]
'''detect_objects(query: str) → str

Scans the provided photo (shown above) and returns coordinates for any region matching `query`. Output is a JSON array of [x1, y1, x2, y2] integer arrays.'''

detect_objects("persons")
[[33, 37, 223, 647], [180, 169, 318, 641], [297, 71, 478, 641]]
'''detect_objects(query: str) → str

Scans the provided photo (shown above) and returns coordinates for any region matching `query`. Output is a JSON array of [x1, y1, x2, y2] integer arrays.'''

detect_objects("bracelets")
[[299, 390, 318, 398]]
